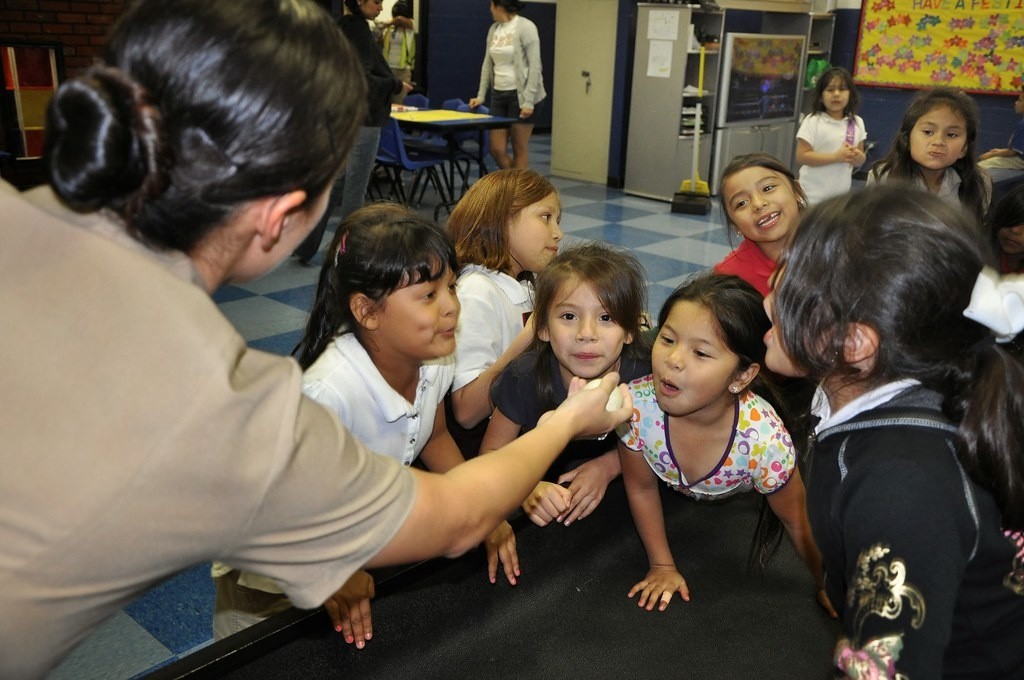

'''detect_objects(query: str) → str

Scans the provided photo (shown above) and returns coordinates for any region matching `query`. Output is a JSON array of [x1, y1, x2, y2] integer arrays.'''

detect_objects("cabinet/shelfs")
[[0, 41, 64, 160], [621, 2, 725, 202], [760, 11, 834, 178], [711, 121, 795, 197]]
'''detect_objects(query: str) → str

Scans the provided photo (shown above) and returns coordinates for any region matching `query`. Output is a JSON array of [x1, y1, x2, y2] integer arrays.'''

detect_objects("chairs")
[[367, 93, 490, 216]]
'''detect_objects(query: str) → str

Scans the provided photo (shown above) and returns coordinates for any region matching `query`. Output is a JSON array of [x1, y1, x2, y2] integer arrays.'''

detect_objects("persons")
[[793, 68, 866, 203], [0, 0, 633, 680], [334, 0, 414, 223], [469, 0, 547, 171], [377, 2, 415, 102], [616, 272, 836, 621], [479, 247, 653, 528], [208, 204, 521, 653], [973, 87, 1024, 362], [866, 88, 992, 223], [443, 170, 562, 463], [762, 183, 1024, 680], [713, 154, 811, 298]]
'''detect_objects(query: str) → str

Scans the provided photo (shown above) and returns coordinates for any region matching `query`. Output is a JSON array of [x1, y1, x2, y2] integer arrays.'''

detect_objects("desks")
[[139, 470, 843, 680], [390, 102, 517, 223]]
